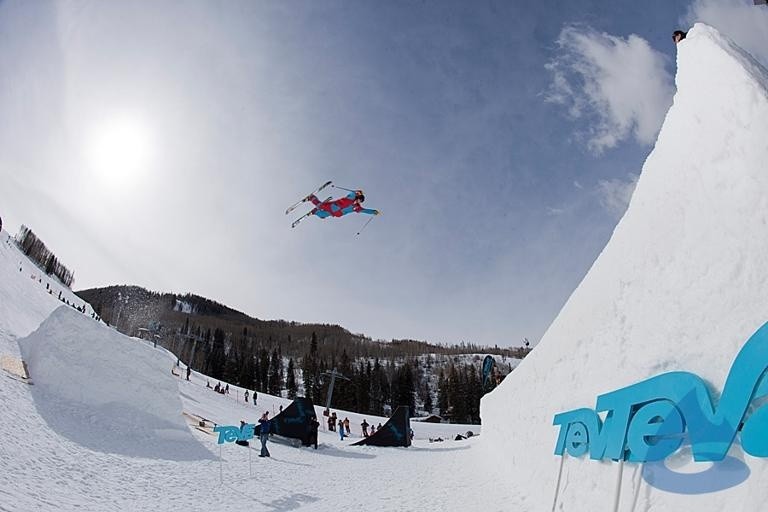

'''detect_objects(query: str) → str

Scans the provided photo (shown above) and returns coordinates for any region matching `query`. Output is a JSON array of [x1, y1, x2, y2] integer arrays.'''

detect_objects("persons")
[[253, 392, 257, 405], [279, 404, 283, 412], [206, 380, 229, 394], [46, 283, 109, 327], [325, 406, 382, 437], [302, 189, 378, 219], [245, 390, 249, 402], [257, 413, 270, 456], [186, 365, 190, 381]]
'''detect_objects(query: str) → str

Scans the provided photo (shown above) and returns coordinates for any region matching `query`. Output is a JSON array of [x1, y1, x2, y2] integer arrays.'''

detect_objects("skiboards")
[[286, 182, 332, 228]]
